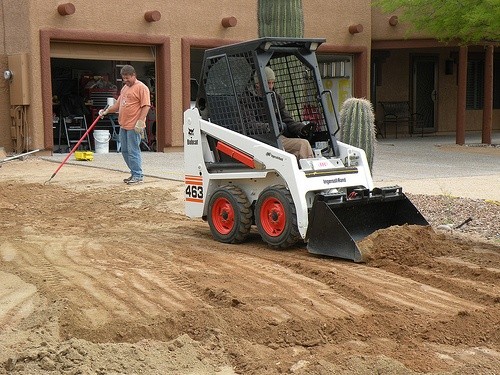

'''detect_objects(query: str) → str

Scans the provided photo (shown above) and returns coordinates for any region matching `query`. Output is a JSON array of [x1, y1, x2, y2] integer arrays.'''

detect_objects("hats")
[[254, 67, 276, 83]]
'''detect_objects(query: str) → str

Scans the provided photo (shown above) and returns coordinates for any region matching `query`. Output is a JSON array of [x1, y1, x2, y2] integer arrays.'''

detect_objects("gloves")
[[98, 109, 108, 119], [135, 120, 145, 133], [275, 122, 287, 132], [302, 123, 311, 137]]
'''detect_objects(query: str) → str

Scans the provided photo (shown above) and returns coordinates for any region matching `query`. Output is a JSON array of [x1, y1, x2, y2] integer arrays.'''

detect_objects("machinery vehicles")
[[184, 38, 430, 262]]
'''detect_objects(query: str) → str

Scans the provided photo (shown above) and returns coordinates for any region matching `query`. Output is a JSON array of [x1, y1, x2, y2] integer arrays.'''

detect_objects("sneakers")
[[124, 177, 143, 184]]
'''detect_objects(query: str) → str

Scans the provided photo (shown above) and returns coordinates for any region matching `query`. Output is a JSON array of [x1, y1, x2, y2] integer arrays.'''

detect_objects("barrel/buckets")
[[92, 130, 111, 153]]
